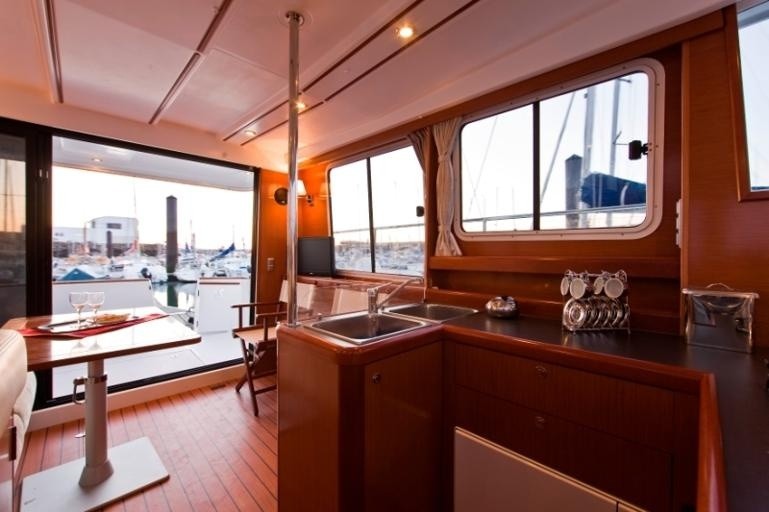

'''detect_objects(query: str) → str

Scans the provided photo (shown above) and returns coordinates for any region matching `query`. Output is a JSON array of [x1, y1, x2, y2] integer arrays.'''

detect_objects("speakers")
[[416, 206, 424, 216], [629, 139, 641, 160]]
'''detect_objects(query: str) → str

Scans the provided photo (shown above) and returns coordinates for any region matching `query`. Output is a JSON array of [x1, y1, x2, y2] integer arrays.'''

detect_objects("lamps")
[[274, 179, 308, 205]]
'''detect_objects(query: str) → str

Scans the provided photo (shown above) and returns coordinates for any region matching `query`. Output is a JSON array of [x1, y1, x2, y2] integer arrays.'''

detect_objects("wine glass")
[[68, 291, 104, 331]]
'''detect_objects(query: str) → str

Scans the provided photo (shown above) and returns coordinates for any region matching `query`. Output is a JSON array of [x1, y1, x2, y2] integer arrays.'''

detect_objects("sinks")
[[305, 312, 432, 344], [382, 301, 481, 325]]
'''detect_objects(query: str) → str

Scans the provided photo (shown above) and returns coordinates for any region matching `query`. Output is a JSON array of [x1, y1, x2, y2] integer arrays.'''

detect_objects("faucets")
[[365, 275, 425, 313]]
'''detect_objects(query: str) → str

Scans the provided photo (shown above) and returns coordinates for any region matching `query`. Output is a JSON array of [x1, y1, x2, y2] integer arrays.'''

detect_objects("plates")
[[561, 297, 629, 333], [87, 314, 130, 323]]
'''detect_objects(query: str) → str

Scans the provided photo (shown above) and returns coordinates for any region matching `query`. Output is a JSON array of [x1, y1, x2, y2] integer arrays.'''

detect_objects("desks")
[[1, 305, 201, 512]]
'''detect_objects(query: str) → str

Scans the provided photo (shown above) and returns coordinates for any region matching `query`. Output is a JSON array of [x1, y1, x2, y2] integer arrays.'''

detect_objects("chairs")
[[276, 285, 391, 325], [0, 328, 37, 512], [231, 279, 318, 417]]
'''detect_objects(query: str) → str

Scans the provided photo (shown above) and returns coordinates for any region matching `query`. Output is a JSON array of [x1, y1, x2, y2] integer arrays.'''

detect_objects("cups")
[[560, 269, 629, 298]]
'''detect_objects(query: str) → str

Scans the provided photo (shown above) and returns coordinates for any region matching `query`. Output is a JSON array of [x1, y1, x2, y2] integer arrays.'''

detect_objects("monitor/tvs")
[[298, 236, 335, 277]]
